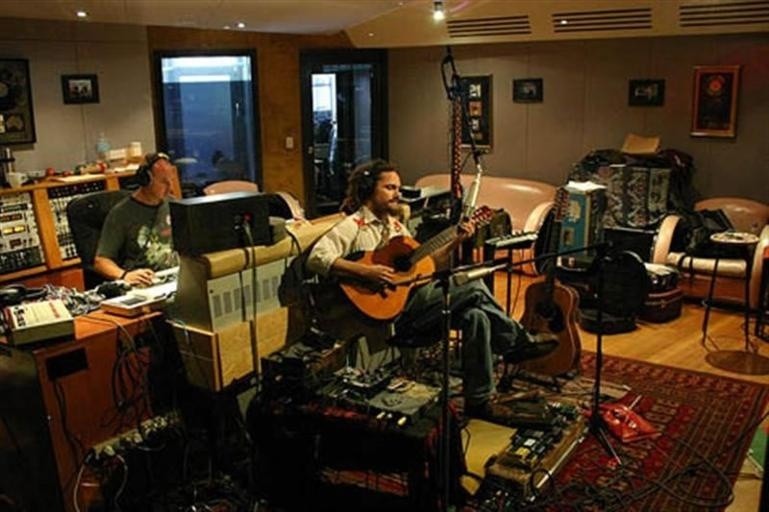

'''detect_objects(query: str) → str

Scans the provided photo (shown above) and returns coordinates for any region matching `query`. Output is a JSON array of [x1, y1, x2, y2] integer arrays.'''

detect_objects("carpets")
[[307, 346, 769, 512]]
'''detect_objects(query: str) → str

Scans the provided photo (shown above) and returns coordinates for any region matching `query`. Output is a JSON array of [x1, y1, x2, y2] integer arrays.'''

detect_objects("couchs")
[[414, 173, 559, 276]]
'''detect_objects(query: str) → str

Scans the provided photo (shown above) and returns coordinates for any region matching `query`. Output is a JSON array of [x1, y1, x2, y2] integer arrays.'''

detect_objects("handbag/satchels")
[[681, 208, 743, 260]]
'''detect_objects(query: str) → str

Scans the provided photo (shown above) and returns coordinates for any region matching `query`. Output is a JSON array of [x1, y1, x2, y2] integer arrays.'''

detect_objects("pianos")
[[485, 230, 537, 250]]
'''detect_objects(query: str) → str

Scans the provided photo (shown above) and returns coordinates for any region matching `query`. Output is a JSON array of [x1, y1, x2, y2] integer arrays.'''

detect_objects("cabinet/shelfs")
[[0, 164, 184, 284]]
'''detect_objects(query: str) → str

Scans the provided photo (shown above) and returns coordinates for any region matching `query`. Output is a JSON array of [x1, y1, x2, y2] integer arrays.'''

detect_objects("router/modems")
[[101, 294, 167, 318]]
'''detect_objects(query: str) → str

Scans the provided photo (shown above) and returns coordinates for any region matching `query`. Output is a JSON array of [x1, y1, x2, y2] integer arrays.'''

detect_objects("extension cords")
[[91, 416, 168, 462]]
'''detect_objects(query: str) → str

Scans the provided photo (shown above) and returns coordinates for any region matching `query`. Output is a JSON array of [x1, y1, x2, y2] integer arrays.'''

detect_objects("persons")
[[523, 83, 535, 97], [636, 83, 658, 99], [701, 75, 728, 124], [308, 161, 560, 426], [94, 152, 199, 288]]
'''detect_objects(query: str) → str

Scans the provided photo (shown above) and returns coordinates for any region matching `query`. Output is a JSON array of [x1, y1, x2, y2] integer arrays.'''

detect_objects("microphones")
[[463, 172, 482, 223], [448, 263, 508, 286]]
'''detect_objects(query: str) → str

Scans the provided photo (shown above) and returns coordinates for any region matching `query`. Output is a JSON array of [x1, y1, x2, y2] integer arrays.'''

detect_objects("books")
[[621, 132, 661, 154], [2, 298, 77, 347]]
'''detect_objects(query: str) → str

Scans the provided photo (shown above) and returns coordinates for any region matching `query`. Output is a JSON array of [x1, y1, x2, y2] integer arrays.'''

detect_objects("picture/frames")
[[689, 64, 742, 139], [0, 57, 38, 145], [513, 77, 544, 103], [460, 73, 494, 149], [60, 73, 100, 105], [624, 72, 673, 113]]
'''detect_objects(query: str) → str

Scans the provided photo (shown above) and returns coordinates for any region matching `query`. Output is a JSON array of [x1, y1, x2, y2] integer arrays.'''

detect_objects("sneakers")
[[505, 332, 559, 365], [464, 402, 512, 419]]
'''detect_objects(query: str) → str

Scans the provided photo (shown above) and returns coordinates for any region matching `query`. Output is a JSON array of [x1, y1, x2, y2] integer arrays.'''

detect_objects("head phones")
[[134, 152, 174, 187], [0, 285, 48, 310], [357, 159, 387, 202]]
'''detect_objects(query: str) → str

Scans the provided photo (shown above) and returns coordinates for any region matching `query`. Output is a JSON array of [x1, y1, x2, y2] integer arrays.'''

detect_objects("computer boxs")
[[168, 191, 272, 257]]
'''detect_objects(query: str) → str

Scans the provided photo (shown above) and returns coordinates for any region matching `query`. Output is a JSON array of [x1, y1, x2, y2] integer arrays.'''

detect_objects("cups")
[[7, 172, 28, 189]]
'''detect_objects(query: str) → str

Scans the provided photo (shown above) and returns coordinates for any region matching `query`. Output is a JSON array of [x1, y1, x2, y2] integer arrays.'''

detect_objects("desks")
[[0, 306, 164, 512], [701, 231, 760, 349]]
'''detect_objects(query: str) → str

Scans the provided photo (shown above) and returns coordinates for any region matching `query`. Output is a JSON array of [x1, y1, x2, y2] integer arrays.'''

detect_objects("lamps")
[[431, 2, 446, 22]]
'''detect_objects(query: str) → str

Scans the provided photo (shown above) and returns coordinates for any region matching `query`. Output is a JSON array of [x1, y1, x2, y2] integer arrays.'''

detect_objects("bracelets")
[[120, 270, 129, 280]]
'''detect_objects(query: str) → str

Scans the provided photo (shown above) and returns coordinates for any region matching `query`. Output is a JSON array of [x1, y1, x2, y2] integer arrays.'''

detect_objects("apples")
[[46, 167, 55, 176]]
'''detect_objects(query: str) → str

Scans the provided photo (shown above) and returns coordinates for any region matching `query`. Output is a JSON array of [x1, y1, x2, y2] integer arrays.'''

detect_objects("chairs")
[[66, 191, 130, 290], [436, 217, 495, 359], [284, 217, 320, 253], [653, 197, 769, 313]]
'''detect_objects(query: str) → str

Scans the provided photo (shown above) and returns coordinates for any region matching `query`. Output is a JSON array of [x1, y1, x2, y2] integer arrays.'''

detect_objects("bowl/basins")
[[27, 168, 46, 181]]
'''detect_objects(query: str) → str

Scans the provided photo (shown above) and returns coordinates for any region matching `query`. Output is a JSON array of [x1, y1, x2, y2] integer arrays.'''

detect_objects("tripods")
[[509, 240, 638, 467]]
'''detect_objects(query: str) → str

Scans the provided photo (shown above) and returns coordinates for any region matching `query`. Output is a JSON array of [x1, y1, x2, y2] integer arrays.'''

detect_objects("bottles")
[[94, 131, 112, 168], [0, 148, 15, 187], [127, 141, 143, 163]]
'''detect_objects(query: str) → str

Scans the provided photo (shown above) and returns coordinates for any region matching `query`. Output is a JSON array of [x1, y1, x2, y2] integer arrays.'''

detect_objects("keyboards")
[[155, 265, 181, 278]]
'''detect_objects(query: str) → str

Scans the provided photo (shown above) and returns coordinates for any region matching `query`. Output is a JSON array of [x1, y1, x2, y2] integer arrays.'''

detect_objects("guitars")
[[337, 204, 494, 325], [513, 187, 581, 377]]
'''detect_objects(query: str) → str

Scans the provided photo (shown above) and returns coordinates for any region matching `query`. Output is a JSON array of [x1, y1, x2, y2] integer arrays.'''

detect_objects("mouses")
[[151, 276, 161, 285]]
